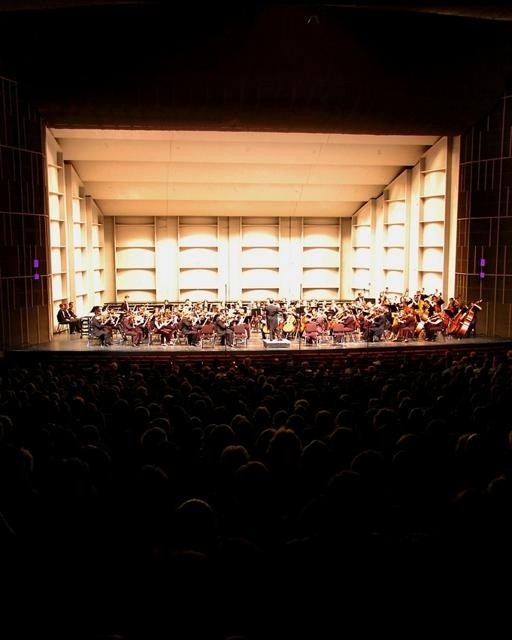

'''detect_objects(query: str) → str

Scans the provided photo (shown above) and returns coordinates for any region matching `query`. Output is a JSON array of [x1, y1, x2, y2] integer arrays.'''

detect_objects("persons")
[[0, 289, 512, 552]]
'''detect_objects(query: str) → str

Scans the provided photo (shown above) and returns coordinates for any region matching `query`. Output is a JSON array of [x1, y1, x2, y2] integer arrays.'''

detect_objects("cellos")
[[381, 286, 483, 339]]
